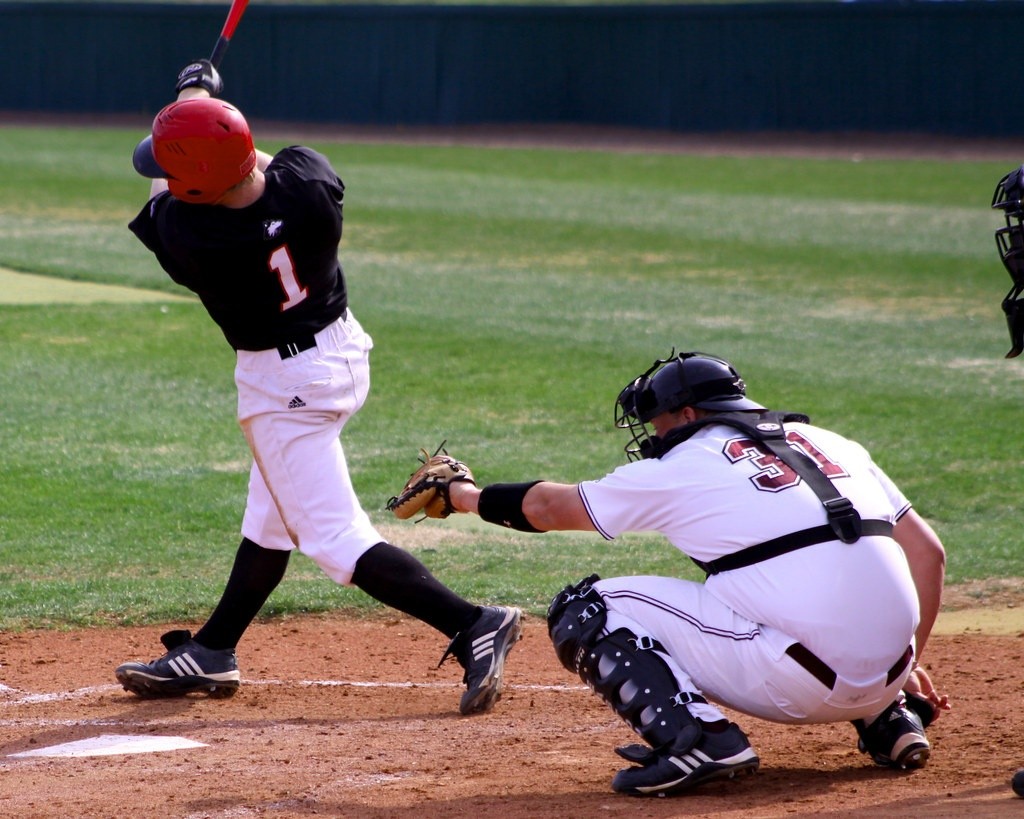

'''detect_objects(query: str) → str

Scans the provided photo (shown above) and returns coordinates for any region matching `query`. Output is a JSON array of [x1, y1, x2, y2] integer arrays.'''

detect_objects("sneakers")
[[612, 722, 759, 797], [863, 703, 930, 769], [438, 605, 522, 715], [116, 629, 240, 698], [1012, 770, 1024, 796]]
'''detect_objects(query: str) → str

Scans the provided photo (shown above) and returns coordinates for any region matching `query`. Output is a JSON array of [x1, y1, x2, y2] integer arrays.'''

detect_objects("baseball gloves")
[[390, 455, 477, 520]]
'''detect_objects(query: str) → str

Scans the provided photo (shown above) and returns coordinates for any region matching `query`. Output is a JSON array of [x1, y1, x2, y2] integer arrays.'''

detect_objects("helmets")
[[132, 98, 256, 205], [646, 351, 769, 423]]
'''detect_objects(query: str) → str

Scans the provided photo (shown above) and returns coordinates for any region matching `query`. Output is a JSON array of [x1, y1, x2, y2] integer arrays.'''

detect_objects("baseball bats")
[[207, 0, 251, 69]]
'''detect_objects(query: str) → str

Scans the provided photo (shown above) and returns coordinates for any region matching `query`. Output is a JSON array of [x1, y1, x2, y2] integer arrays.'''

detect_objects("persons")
[[114, 59, 523, 714], [387, 347, 951, 800]]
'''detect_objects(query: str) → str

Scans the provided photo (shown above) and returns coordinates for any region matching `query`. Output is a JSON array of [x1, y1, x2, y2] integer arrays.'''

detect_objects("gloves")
[[175, 59, 224, 96]]
